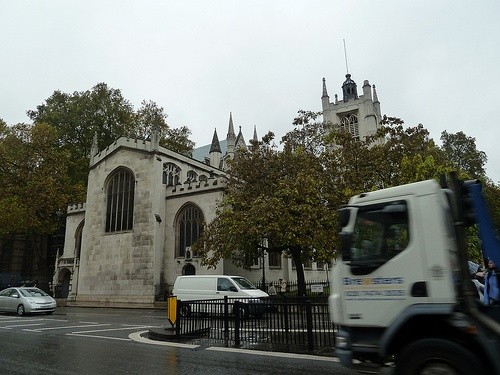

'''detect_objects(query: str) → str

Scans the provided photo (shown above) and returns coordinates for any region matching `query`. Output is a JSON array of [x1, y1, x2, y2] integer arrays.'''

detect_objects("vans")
[[172, 274, 270, 320]]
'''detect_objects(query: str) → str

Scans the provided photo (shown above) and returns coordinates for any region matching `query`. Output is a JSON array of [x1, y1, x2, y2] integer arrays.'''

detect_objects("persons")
[[468, 259, 499, 305], [279, 278, 287, 292], [268, 282, 276, 295]]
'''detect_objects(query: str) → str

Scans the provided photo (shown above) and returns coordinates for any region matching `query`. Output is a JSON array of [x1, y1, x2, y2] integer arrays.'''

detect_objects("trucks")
[[327, 171, 500, 375]]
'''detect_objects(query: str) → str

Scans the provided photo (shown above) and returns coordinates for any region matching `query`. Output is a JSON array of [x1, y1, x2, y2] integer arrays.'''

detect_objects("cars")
[[0, 287, 57, 316]]
